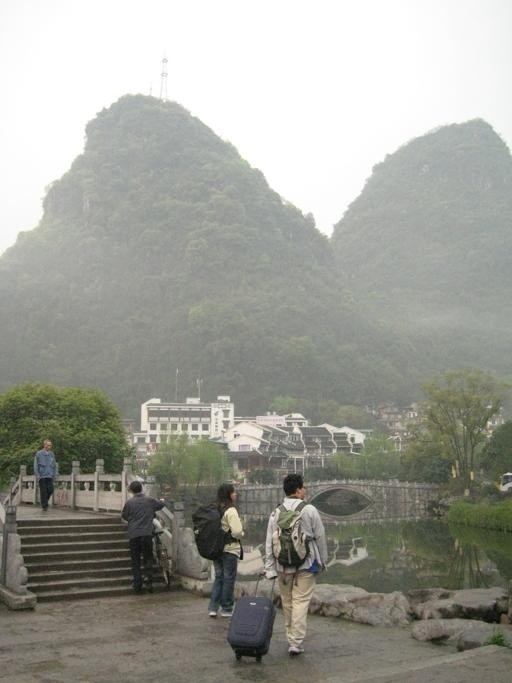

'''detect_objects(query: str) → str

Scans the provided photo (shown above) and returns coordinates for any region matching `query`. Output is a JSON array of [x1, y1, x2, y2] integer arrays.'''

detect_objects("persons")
[[34, 438, 58, 511], [120, 482, 165, 592], [264, 473, 328, 655], [207, 483, 245, 618]]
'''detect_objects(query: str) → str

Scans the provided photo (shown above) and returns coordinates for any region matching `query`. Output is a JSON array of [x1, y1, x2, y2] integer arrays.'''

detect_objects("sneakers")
[[288, 646, 305, 654], [221, 611, 233, 617], [209, 611, 217, 617]]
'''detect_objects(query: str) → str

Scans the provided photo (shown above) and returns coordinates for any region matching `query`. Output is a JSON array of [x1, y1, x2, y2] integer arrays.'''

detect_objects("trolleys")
[[229, 570, 285, 661]]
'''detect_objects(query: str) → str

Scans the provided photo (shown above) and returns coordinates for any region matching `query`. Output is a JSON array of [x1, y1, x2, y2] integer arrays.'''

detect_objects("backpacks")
[[272, 502, 310, 567], [192, 505, 240, 560]]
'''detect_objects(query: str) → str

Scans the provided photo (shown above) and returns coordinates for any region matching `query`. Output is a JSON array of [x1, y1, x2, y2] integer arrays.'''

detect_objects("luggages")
[[228, 573, 277, 663]]
